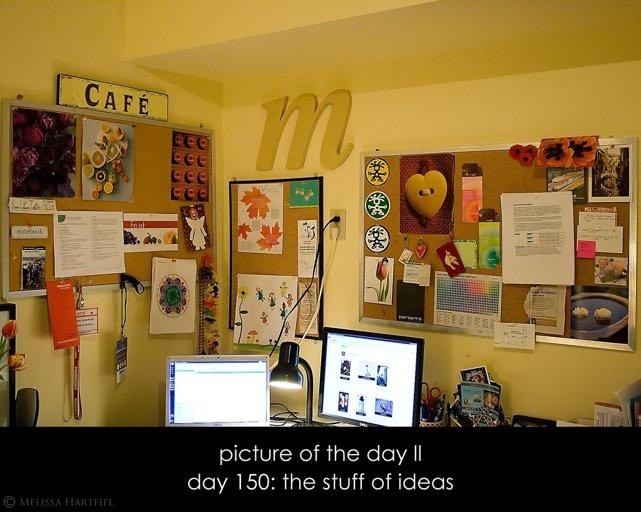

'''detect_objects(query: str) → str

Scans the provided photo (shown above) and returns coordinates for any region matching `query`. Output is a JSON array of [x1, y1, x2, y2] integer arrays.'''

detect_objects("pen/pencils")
[[421, 394, 445, 423], [447, 402, 450, 427]]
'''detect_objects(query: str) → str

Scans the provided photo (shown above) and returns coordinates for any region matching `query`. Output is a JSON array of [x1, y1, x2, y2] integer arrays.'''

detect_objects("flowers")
[[1, 320, 28, 381]]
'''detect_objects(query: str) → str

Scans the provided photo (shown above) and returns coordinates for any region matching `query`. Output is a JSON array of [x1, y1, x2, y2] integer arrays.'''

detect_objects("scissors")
[[421, 382, 441, 418]]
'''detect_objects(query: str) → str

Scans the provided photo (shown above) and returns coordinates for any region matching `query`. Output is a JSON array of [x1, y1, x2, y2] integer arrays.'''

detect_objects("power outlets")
[[329, 208, 346, 240]]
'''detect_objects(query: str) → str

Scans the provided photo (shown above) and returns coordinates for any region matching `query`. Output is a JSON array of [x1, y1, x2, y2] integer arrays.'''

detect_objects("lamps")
[[269, 341, 313, 427]]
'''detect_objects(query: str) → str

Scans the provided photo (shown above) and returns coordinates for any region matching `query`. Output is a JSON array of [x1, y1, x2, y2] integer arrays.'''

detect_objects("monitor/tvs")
[[165, 355, 270, 427], [318, 327, 425, 428]]
